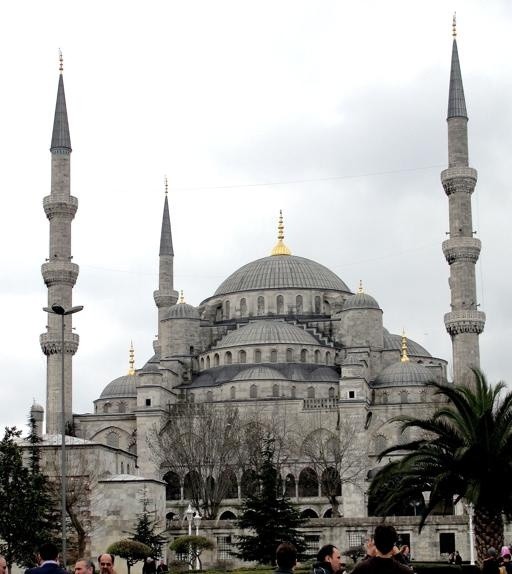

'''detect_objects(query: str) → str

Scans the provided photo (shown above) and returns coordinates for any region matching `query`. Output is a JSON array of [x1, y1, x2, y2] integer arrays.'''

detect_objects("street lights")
[[42, 302, 84, 569], [185, 504, 202, 570], [465, 502, 479, 565]]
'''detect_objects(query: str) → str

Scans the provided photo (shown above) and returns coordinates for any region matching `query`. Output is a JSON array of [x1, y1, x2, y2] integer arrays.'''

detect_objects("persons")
[[446, 543, 511, 573], [349, 525, 417, 574], [400, 544, 412, 563], [142, 556, 154, 574], [74, 557, 96, 574], [23, 543, 66, 574], [157, 559, 169, 574], [313, 544, 342, 574], [96, 553, 118, 574], [273, 543, 299, 573], [0, 554, 7, 574]]
